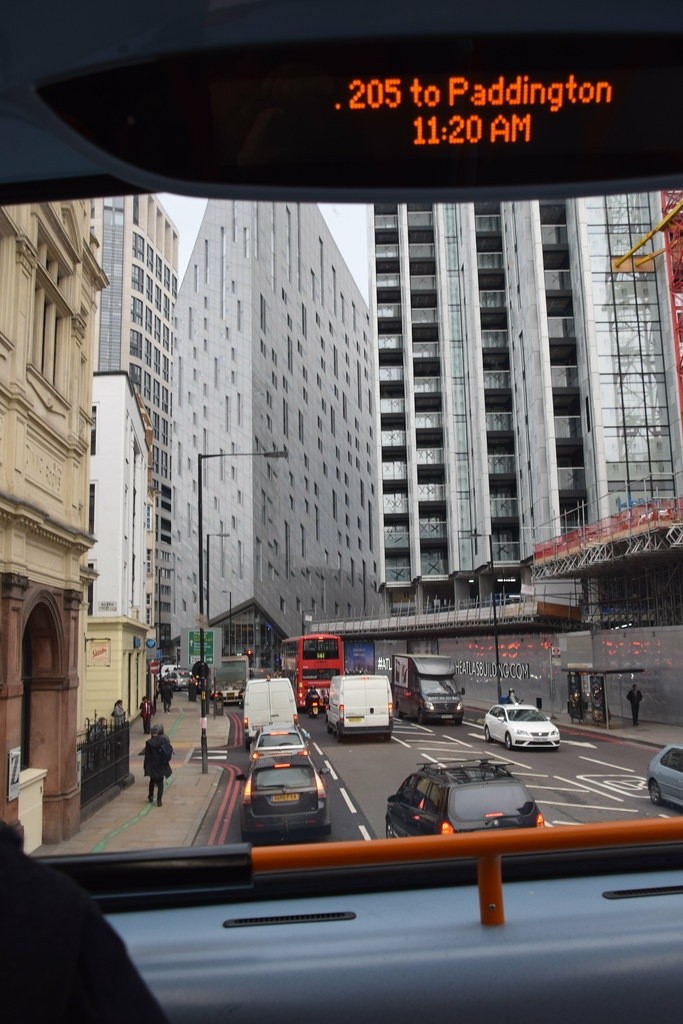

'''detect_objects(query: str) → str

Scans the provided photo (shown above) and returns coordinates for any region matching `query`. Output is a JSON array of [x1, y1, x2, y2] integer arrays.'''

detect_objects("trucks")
[[392, 653, 466, 727]]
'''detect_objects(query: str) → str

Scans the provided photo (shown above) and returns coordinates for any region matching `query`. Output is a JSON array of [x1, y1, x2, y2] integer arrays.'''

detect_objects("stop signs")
[[150, 661, 161, 674]]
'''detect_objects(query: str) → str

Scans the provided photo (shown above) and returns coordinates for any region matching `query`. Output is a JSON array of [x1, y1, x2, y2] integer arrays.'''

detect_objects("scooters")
[[304, 693, 321, 719]]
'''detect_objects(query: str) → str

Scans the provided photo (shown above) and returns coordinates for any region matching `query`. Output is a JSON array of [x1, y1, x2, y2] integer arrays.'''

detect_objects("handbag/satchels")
[[111, 711, 115, 716]]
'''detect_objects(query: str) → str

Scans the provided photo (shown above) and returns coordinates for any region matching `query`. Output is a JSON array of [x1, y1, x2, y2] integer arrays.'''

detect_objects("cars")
[[250, 667, 277, 679], [246, 725, 311, 763], [484, 702, 560, 751], [646, 743, 683, 809], [155, 664, 193, 692]]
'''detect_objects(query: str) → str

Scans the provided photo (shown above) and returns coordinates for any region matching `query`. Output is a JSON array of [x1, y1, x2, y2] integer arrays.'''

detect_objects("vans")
[[323, 674, 393, 742], [240, 678, 298, 751]]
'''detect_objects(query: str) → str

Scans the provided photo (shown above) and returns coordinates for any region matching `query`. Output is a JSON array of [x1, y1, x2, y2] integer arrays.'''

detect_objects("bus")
[[278, 634, 344, 708], [210, 654, 249, 703]]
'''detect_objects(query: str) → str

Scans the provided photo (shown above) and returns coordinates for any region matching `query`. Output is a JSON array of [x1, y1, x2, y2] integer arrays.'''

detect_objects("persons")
[[0, 820, 170, 1024], [161, 677, 173, 712], [91, 717, 105, 740], [627, 684, 642, 726], [140, 697, 154, 733], [144, 724, 173, 805], [507, 687, 521, 704], [305, 685, 320, 714], [114, 700, 125, 748]]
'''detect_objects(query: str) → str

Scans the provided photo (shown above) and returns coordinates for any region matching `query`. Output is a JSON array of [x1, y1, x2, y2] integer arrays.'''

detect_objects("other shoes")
[[157, 798, 162, 806], [167, 708, 170, 712], [148, 795, 154, 802]]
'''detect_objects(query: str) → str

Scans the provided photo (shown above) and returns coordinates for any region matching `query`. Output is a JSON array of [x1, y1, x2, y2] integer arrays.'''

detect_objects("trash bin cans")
[[215, 696, 224, 716]]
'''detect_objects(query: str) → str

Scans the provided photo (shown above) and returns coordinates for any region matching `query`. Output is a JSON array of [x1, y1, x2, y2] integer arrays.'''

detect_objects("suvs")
[[385, 756, 544, 840], [235, 754, 331, 843]]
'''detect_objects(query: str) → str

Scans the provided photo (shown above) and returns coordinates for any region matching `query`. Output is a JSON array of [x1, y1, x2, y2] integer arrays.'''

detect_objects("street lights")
[[158, 567, 176, 650], [470, 533, 503, 701], [205, 532, 230, 716], [197, 451, 289, 774], [222, 590, 231, 656]]
